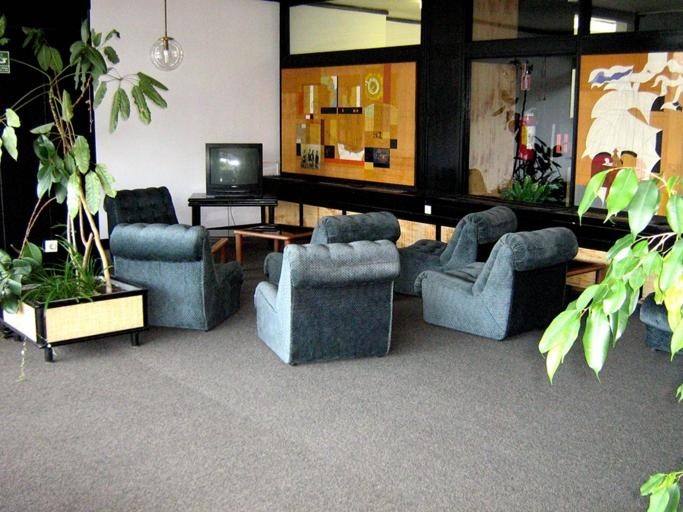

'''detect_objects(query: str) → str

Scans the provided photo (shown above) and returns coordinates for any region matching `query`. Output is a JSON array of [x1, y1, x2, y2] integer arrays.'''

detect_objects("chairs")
[[394, 206, 517, 296], [263, 211, 400, 285], [103, 186, 179, 261], [254, 240, 400, 365], [110, 223, 243, 331], [639, 292, 683, 355], [414, 227, 578, 340]]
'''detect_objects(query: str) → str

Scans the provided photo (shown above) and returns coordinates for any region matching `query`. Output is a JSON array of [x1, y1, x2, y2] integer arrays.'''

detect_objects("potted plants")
[[0, 14, 169, 364]]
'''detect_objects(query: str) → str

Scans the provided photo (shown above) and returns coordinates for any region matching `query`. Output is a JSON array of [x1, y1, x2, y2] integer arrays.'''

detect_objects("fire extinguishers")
[[520, 106, 536, 160]]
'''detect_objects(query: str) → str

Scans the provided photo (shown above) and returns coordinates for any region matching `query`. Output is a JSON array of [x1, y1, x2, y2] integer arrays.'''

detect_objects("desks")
[[187, 192, 277, 226]]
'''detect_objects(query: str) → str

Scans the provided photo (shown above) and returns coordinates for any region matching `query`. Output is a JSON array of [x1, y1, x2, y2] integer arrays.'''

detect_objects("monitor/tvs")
[[205, 142, 263, 198]]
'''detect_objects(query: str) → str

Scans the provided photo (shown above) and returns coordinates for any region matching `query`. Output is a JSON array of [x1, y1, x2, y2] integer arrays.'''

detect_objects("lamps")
[[150, 1, 183, 71]]
